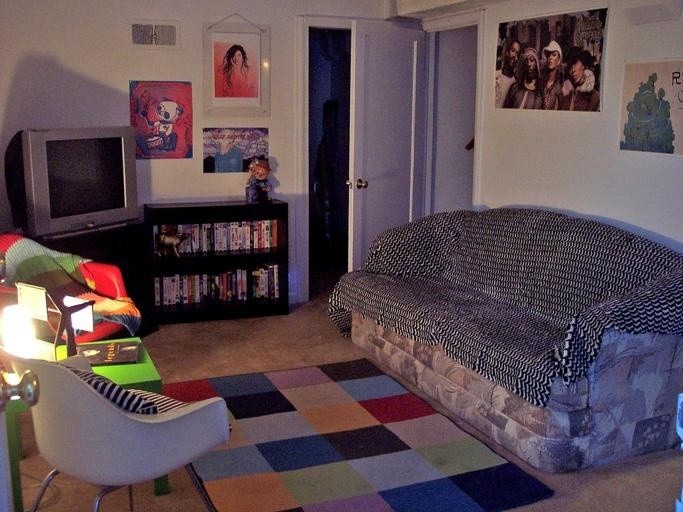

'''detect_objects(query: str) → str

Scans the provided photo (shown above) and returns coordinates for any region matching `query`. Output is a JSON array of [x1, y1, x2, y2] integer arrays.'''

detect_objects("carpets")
[[160, 357, 554, 510]]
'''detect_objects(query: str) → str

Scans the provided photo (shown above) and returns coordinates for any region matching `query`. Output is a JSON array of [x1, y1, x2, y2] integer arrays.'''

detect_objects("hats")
[[543, 40, 563, 57], [565, 46, 585, 61], [519, 47, 541, 78]]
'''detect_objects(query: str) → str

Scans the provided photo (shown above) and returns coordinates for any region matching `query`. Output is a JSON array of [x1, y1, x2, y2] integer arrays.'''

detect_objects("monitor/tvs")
[[5, 127, 140, 239]]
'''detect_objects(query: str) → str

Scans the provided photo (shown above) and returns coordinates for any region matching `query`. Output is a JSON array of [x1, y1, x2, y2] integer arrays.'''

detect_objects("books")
[[76, 341, 140, 367], [154, 219, 279, 306]]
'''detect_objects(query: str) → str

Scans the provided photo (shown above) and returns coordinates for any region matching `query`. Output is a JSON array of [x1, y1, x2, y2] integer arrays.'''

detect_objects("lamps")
[[1, 305, 35, 374]]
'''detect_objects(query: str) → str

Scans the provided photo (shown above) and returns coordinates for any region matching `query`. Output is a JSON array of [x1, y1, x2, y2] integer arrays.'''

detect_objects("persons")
[[495, 41, 521, 108], [561, 46, 600, 111], [216, 43, 257, 96], [502, 47, 544, 109], [540, 41, 565, 110], [247, 160, 273, 200]]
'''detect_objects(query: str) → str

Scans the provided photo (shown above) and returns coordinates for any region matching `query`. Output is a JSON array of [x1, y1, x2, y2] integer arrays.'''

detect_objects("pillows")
[[50, 360, 158, 414]]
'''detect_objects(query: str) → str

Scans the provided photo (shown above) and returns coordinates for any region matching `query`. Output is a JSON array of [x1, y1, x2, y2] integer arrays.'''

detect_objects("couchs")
[[327, 205, 682, 475]]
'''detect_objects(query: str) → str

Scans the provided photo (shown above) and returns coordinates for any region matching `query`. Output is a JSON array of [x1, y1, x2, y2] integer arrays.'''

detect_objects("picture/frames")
[[125, 20, 180, 51], [200, 20, 270, 120], [493, 4, 610, 115], [617, 58, 682, 158]]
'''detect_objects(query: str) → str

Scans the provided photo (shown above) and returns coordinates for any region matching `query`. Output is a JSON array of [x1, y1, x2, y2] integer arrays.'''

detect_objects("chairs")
[[8, 352, 233, 511], [0, 234, 141, 336]]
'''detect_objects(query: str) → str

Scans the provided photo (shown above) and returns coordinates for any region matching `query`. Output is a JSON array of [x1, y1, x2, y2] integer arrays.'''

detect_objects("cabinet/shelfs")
[[52, 224, 160, 339], [145, 197, 291, 320]]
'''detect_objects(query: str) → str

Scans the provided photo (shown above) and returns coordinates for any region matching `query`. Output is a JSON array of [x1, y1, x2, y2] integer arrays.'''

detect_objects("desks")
[[4, 336, 169, 511]]
